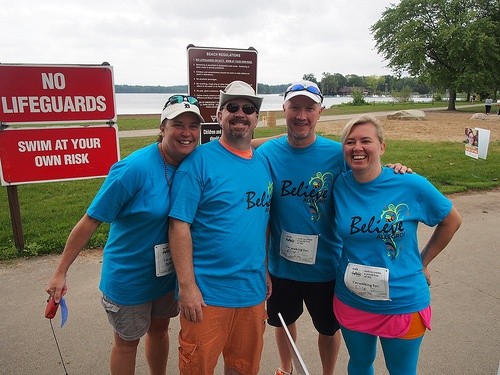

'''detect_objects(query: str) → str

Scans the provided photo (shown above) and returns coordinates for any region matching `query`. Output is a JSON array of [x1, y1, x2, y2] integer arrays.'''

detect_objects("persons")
[[256, 81, 413, 375], [333, 115, 462, 375], [169, 80, 274, 375], [485, 97, 492, 115], [467, 129, 475, 148], [46, 95, 289, 375]]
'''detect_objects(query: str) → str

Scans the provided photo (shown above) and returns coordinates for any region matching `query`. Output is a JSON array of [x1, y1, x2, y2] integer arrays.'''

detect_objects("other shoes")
[[275, 361, 294, 375]]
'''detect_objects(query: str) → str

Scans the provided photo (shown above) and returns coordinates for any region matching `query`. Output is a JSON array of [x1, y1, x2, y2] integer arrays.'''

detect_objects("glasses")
[[285, 84, 323, 103], [221, 103, 259, 115], [163, 95, 200, 110]]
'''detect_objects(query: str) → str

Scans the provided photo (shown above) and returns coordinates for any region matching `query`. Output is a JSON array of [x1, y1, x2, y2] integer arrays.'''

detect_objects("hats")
[[215, 80, 263, 127], [284, 80, 323, 104], [161, 94, 205, 123]]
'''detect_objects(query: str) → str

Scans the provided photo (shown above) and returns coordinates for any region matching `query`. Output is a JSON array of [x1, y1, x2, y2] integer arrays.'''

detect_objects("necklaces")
[[160, 143, 177, 188]]
